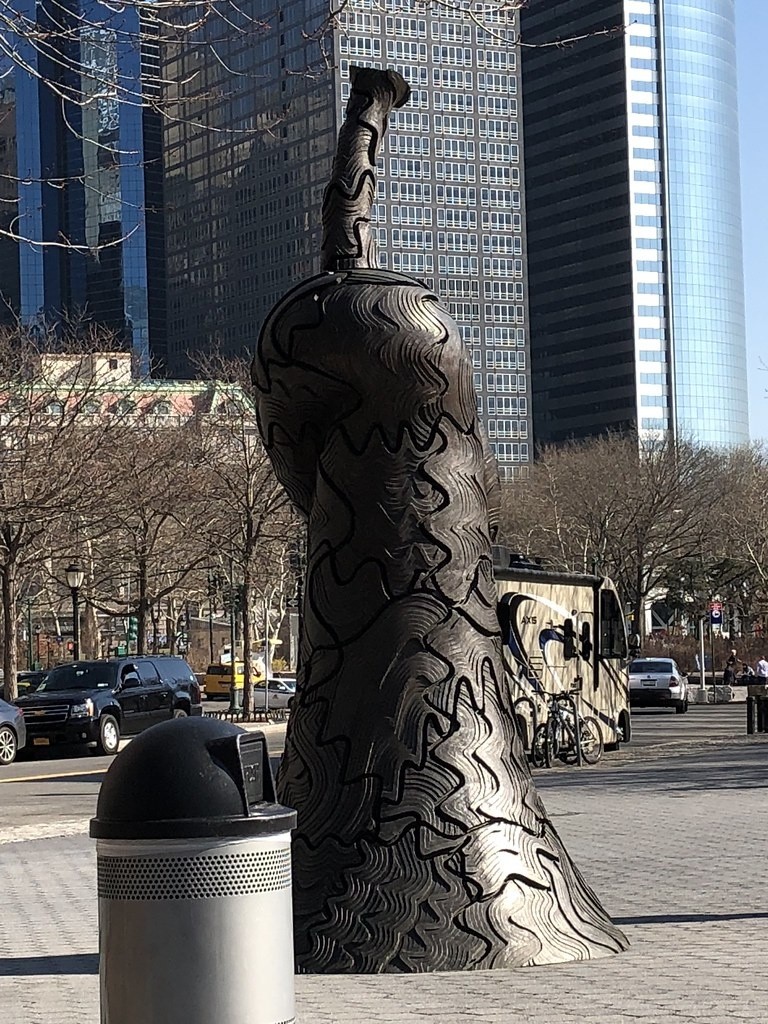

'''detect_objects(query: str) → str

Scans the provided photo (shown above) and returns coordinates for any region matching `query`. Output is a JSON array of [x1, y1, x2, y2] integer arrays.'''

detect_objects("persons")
[[742, 663, 754, 675], [756, 656, 768, 677], [726, 649, 742, 685]]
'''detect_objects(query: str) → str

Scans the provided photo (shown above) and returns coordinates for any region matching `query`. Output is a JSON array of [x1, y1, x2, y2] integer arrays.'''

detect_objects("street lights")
[[65, 564, 85, 661]]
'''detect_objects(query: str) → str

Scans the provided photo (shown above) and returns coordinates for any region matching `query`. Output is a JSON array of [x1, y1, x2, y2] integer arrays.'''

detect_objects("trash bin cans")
[[87, 716, 301, 1024]]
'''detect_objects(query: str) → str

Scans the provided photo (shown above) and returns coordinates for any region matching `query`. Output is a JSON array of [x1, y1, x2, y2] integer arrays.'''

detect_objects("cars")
[[0, 698, 26, 765], [238, 678, 296, 711], [629, 657, 689, 714]]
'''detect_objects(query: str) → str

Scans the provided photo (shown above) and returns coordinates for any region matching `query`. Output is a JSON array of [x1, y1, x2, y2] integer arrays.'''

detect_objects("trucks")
[[493, 554, 639, 764]]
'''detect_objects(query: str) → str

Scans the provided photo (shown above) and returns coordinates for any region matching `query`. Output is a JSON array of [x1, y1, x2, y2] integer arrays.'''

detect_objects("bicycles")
[[531, 688, 604, 768]]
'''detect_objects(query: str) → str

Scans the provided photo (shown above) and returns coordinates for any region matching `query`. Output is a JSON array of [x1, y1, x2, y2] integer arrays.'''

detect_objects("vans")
[[204, 661, 265, 701], [10, 654, 203, 755]]
